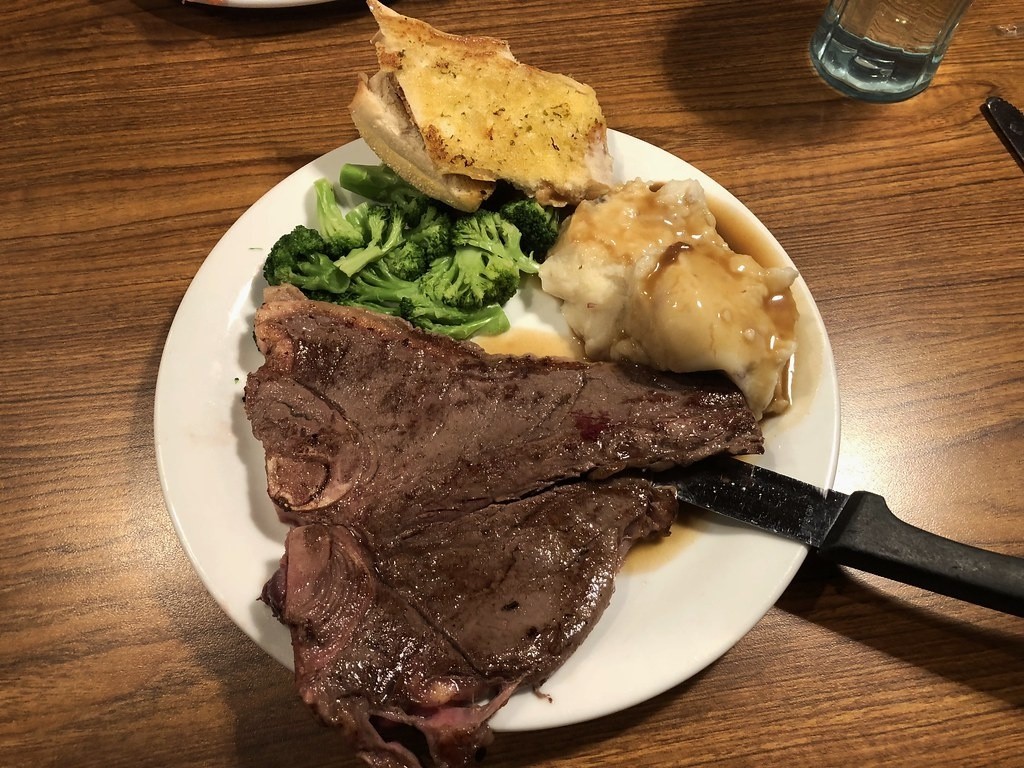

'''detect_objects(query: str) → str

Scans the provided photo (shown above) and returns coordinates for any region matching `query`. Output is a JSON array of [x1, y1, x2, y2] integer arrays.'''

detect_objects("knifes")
[[985, 94, 1024, 167], [680, 457, 1024, 627]]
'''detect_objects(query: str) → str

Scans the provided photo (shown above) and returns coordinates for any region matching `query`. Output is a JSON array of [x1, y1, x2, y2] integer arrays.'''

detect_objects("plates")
[[151, 125, 843, 736]]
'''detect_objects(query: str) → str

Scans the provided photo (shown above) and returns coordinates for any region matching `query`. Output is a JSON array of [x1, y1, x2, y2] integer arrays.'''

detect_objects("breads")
[[348, 0, 613, 212]]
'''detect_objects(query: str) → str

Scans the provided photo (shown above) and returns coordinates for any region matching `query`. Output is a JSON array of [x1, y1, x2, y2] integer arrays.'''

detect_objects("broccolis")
[[262, 163, 565, 344]]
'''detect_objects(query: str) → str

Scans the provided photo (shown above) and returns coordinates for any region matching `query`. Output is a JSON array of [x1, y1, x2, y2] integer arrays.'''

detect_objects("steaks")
[[239, 283, 764, 768]]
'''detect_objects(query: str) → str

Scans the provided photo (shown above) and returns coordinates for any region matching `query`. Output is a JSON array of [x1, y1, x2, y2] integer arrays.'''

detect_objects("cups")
[[807, 0, 976, 104]]
